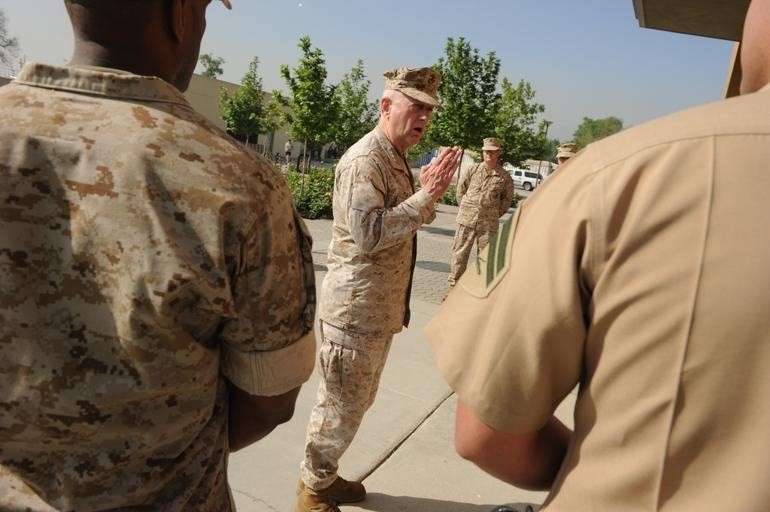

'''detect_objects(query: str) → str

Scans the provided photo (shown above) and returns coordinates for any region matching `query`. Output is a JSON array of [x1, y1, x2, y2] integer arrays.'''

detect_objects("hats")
[[222, 0, 232, 10], [556, 143, 578, 158], [383, 67, 445, 112], [482, 138, 500, 150]]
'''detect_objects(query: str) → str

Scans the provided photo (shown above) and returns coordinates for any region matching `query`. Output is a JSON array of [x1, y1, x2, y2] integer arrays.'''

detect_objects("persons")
[[555, 143, 578, 165], [1, 2, 316, 511], [426, 0, 770, 512], [443, 136, 516, 301], [291, 66, 464, 512], [284, 139, 292, 164]]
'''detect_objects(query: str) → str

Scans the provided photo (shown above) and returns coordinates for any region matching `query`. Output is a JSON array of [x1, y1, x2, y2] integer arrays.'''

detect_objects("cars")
[[505, 167, 542, 192]]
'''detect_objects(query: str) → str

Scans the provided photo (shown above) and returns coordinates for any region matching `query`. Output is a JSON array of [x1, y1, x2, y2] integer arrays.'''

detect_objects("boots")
[[296, 476, 366, 512]]
